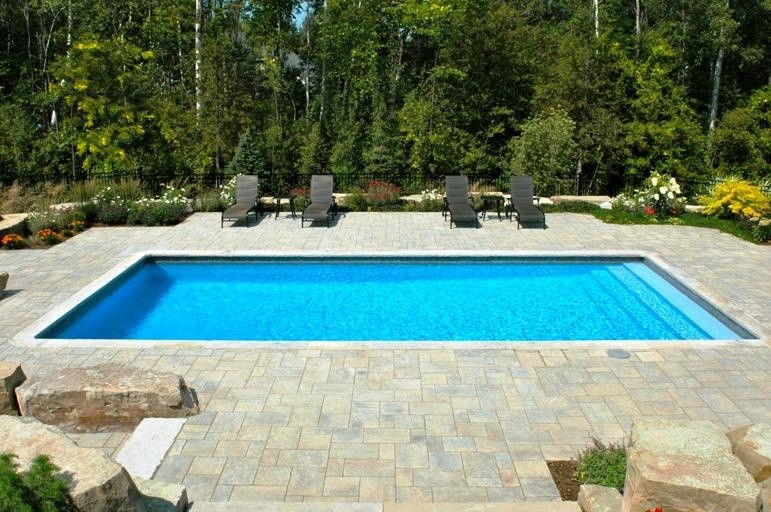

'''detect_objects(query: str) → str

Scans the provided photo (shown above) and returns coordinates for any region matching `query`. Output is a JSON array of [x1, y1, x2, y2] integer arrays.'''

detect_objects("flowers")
[[635, 168, 687, 210]]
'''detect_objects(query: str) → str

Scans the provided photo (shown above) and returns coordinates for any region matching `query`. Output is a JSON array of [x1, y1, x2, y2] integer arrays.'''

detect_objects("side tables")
[[480, 195, 504, 221], [273, 195, 298, 220]]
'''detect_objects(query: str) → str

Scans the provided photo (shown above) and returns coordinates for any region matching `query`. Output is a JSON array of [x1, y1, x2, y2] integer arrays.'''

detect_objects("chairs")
[[441, 175, 479, 230], [301, 174, 338, 228], [221, 174, 263, 228], [506, 175, 546, 230]]
